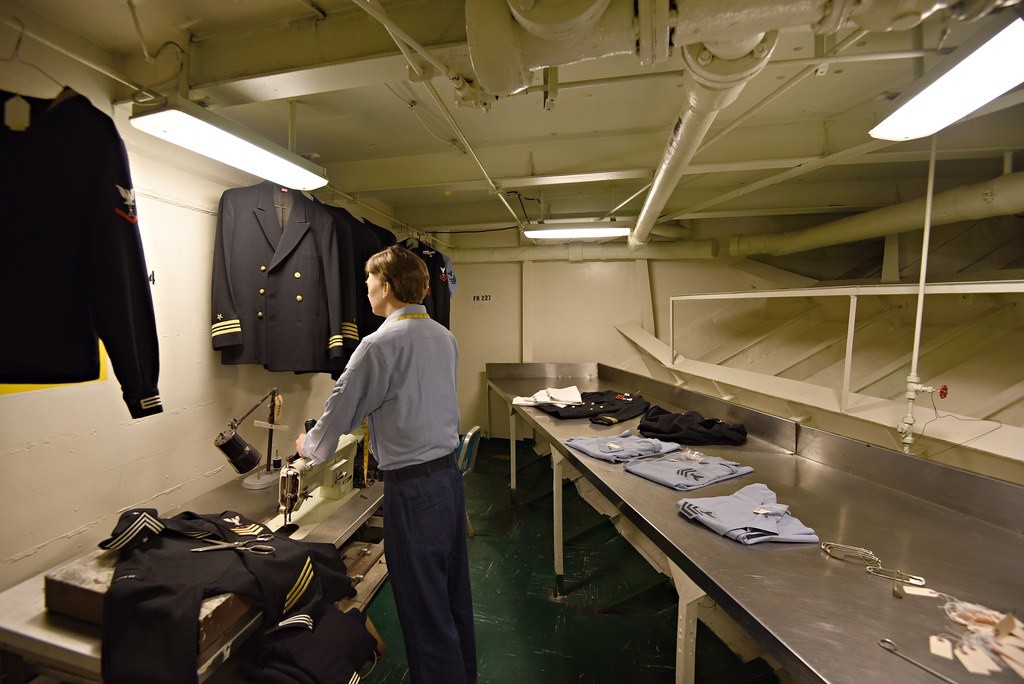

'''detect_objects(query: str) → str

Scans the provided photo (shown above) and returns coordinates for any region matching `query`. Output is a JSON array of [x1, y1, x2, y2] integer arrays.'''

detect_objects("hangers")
[[274, 186, 453, 249], [0, 14, 118, 130]]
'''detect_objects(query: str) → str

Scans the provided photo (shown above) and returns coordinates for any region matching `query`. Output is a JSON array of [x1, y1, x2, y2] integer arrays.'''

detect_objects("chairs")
[[449, 425, 482, 538]]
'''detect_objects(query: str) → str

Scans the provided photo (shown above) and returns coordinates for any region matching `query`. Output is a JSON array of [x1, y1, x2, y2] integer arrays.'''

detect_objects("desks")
[[483, 361, 1024, 684]]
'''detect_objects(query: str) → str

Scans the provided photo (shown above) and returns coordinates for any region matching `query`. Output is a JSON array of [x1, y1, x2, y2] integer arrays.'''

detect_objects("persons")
[[294, 245, 479, 684]]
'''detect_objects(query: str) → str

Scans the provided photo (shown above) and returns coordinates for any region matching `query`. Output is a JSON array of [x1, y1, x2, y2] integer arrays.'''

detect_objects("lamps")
[[867, 14, 1024, 142], [523, 188, 632, 240], [129, 25, 329, 192], [214, 385, 280, 490]]
